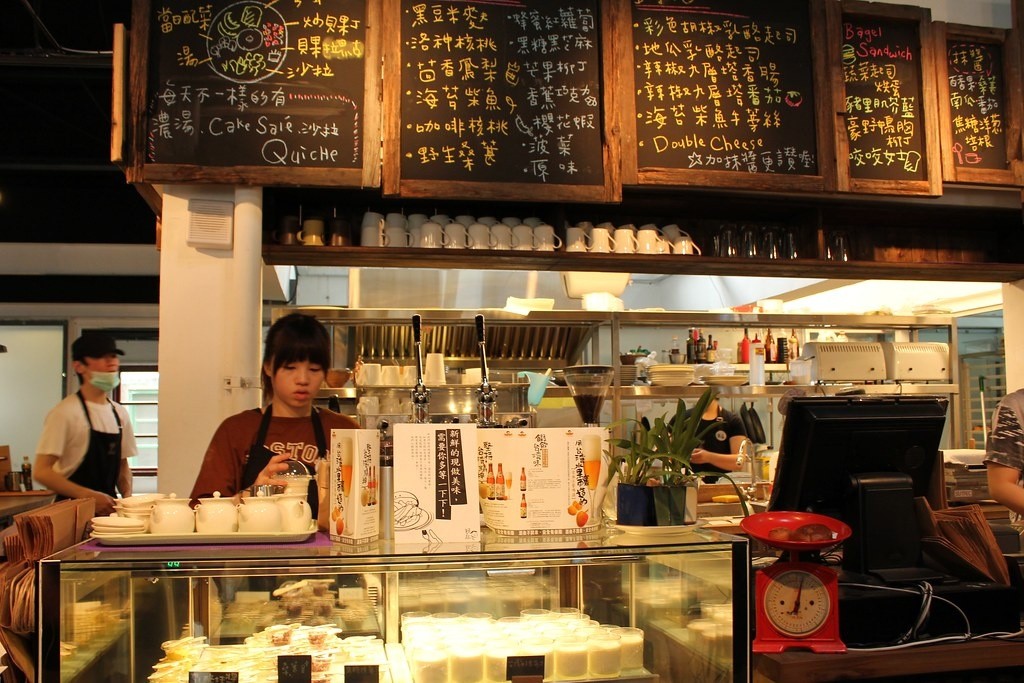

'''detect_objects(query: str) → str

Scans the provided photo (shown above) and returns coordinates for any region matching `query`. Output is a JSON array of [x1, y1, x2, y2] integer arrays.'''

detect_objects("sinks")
[[691, 503, 768, 523]]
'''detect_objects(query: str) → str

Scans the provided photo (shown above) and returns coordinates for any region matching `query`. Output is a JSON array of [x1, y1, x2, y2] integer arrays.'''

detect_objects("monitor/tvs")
[[766, 393, 959, 588]]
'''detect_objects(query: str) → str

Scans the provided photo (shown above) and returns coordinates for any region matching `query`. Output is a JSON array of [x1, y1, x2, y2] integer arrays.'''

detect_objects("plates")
[[89, 494, 165, 537], [549, 364, 750, 387]]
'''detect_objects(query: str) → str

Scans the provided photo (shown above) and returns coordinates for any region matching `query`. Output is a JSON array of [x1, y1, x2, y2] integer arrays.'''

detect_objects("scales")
[[740, 512, 852, 654]]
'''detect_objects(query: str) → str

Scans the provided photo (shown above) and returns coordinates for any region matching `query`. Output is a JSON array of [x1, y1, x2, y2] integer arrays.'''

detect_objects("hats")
[[71, 333, 125, 362]]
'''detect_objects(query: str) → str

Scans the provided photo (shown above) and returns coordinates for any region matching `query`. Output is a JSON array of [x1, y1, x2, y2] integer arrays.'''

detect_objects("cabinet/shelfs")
[[34, 522, 749, 683], [260, 243, 1024, 517]]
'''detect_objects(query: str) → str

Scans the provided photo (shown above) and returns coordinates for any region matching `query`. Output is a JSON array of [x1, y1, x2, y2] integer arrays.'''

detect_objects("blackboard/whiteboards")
[[124, 0, 382, 191], [379, 0, 622, 202], [823, 0, 944, 197], [613, 0, 841, 192], [932, 20, 1024, 186]]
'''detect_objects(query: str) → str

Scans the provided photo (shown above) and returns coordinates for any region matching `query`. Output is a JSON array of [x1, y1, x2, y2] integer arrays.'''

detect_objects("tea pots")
[[148, 491, 312, 535]]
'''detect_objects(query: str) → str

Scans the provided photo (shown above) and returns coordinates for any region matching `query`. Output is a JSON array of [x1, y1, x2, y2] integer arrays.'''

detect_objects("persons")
[[982, 387, 1024, 533], [191, 314, 363, 602], [35, 332, 139, 516], [647, 396, 747, 486]]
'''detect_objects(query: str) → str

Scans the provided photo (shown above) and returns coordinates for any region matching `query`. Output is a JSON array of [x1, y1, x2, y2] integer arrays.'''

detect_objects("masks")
[[81, 364, 119, 391]]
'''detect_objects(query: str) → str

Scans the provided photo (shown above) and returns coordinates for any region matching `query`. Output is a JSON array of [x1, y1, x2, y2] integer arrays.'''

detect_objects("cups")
[[297, 212, 701, 261], [790, 361, 811, 385], [5, 471, 22, 492], [400, 607, 644, 682], [271, 475, 312, 502], [464, 366, 491, 385], [361, 354, 447, 386]]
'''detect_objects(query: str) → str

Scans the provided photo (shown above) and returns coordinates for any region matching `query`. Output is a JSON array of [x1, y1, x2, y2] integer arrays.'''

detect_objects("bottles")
[[520, 494, 527, 518], [662, 350, 672, 363], [670, 335, 680, 354], [686, 329, 799, 385], [367, 467, 376, 506], [487, 464, 494, 499], [22, 455, 34, 492], [496, 464, 506, 499], [520, 468, 527, 491]]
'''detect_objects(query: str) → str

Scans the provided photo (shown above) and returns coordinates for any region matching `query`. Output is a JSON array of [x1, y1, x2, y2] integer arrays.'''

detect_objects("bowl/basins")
[[669, 354, 687, 365], [327, 368, 352, 387], [621, 354, 649, 365]]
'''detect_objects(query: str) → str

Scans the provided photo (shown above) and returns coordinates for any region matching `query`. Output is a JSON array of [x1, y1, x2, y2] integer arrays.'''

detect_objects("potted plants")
[[655, 388, 749, 524], [603, 412, 669, 526]]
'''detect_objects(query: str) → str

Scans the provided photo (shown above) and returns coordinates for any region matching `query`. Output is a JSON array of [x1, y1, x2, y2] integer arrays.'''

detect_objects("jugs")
[[713, 224, 802, 260], [824, 229, 853, 263]]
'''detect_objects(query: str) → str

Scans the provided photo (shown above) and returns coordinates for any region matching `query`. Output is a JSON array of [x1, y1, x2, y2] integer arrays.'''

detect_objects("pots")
[[730, 398, 766, 444]]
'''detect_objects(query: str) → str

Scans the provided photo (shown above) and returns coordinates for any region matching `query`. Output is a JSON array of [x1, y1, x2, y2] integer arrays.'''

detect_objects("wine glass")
[[341, 439, 353, 536], [582, 435, 601, 525], [506, 472, 512, 500]]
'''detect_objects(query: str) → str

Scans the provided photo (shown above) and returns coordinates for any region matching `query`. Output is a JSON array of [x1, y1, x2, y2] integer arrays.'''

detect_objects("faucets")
[[736, 435, 758, 498]]
